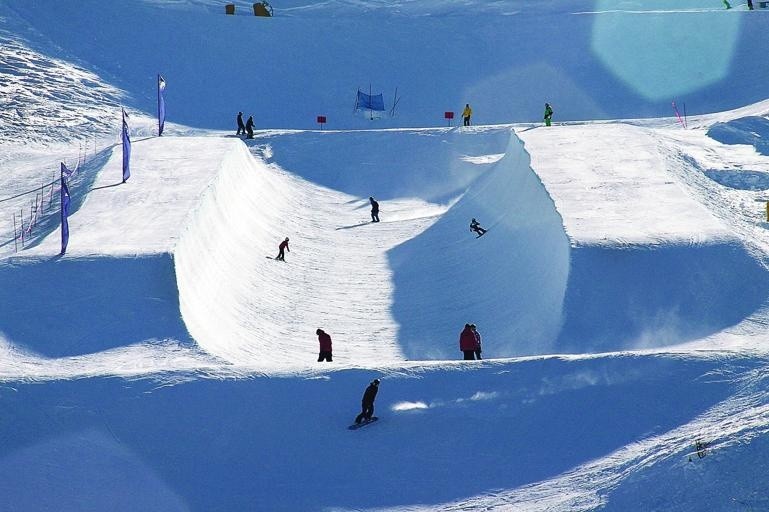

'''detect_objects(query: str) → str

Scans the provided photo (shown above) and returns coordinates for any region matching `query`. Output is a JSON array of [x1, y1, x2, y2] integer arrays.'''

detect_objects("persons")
[[235, 111, 247, 135], [543, 102, 553, 127], [245, 116, 256, 139], [315, 328, 334, 362], [355, 378, 382, 424], [460, 104, 472, 127], [369, 196, 380, 222], [469, 217, 487, 236], [274, 236, 290, 263], [469, 324, 483, 360], [459, 323, 479, 360]]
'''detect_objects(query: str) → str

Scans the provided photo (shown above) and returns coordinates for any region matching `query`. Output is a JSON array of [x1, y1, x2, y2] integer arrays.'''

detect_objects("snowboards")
[[348, 417, 378, 430], [476, 229, 489, 238], [266, 257, 288, 263]]
